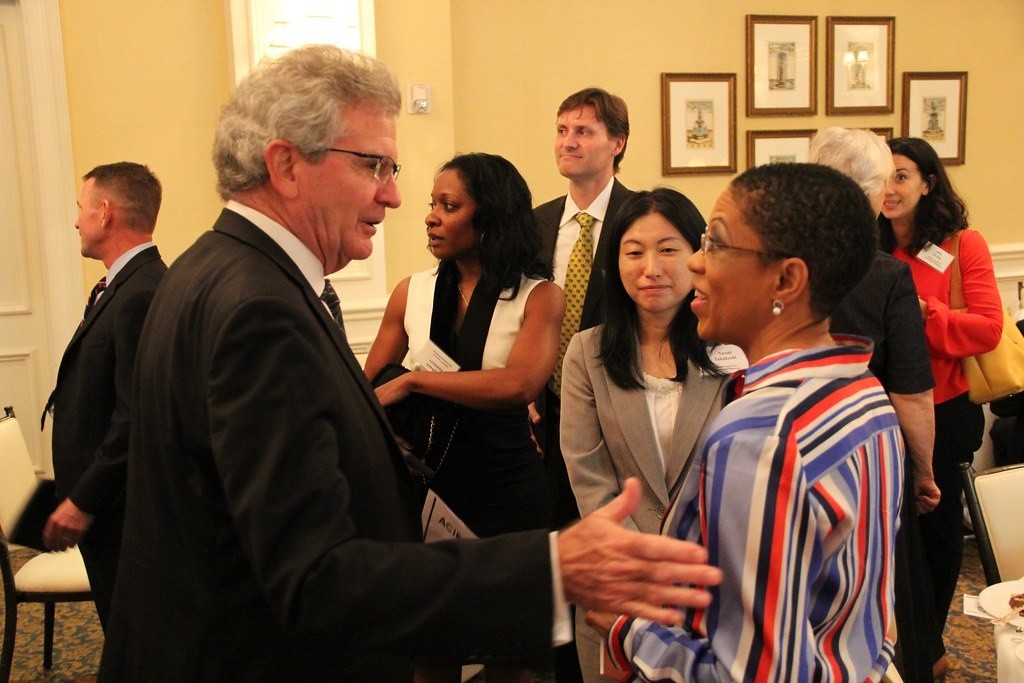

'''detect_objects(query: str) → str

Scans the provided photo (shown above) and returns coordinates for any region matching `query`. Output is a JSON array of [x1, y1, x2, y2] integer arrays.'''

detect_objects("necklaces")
[[459, 290, 470, 306]]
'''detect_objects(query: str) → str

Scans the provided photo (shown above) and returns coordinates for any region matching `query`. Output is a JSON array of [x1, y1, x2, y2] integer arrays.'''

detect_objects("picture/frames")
[[744, 129, 818, 171], [847, 127, 893, 144], [660, 72, 738, 176], [900, 71, 968, 166], [826, 15, 896, 116], [745, 14, 818, 117]]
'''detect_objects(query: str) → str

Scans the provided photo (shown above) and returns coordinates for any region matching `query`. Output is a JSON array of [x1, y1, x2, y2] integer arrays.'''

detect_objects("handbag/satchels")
[[950, 229, 1024, 405]]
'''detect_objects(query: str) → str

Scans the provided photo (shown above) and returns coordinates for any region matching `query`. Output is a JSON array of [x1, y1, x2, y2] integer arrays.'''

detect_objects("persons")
[[802, 125, 942, 520], [557, 188, 749, 539], [584, 164, 906, 683], [44, 162, 169, 638], [521, 88, 646, 447], [365, 152, 580, 547], [96, 45, 727, 683], [874, 135, 1005, 683]]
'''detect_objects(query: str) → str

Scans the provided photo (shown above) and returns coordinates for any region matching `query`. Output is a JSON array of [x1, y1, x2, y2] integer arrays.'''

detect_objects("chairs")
[[959, 460, 1024, 587], [0, 405, 93, 683]]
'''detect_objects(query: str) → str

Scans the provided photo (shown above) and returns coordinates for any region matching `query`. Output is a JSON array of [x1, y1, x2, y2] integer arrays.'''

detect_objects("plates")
[[979, 580, 1024, 630]]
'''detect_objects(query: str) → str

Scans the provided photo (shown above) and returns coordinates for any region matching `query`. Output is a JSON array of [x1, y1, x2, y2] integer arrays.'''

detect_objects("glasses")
[[311, 149, 402, 183], [700, 226, 816, 284]]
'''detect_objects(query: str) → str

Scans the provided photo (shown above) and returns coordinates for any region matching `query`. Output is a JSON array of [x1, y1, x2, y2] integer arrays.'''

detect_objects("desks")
[[994, 578, 1024, 683]]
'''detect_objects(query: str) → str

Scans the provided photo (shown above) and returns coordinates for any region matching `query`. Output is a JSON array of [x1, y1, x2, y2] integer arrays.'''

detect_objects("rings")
[[63, 535, 72, 543]]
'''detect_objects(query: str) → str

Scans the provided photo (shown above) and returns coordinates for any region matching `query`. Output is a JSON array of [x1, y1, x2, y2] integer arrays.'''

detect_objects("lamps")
[[844, 50, 871, 89]]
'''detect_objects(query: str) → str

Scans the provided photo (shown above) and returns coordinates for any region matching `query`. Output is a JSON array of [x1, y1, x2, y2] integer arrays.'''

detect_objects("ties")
[[320, 279, 346, 340], [73, 277, 108, 335], [548, 213, 597, 400]]
